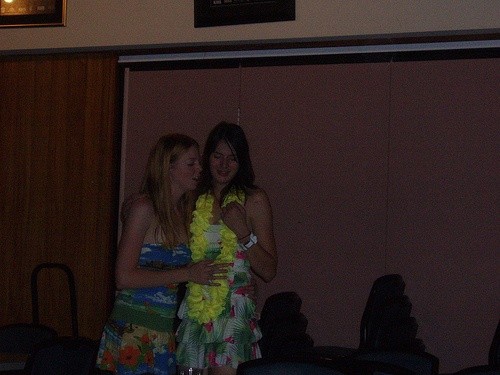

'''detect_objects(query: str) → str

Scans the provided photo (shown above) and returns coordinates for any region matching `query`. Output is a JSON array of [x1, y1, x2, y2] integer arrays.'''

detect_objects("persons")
[[175, 122, 278, 375], [96, 133, 258, 375]]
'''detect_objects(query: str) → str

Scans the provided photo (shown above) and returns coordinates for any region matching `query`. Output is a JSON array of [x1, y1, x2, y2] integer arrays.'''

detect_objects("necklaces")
[[184, 184, 246, 322]]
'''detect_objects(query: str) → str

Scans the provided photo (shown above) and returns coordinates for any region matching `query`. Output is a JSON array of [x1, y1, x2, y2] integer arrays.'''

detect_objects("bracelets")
[[239, 232, 254, 241], [244, 233, 257, 249]]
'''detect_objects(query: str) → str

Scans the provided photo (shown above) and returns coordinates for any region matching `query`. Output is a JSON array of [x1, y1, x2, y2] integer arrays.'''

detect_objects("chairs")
[[0, 322, 440, 375]]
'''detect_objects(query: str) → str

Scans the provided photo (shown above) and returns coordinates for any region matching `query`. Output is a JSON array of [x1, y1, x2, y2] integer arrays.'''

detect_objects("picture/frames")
[[0, 0, 67, 27]]
